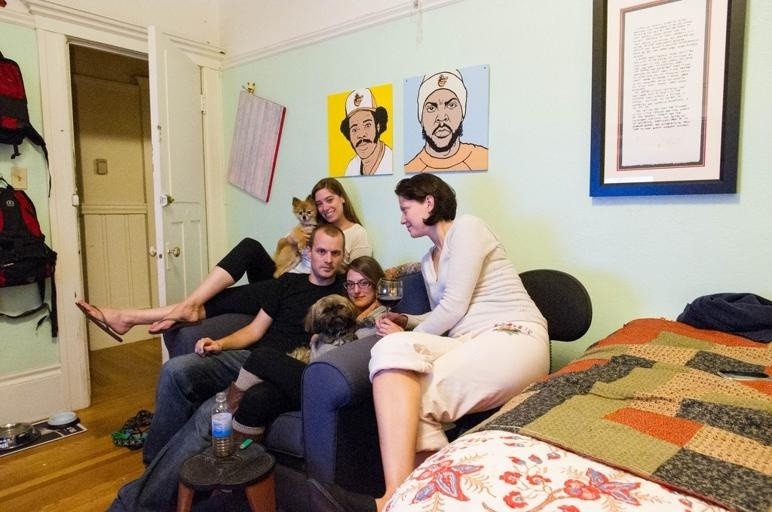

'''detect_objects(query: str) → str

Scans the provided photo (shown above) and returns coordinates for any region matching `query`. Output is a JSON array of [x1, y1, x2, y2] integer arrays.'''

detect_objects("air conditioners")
[[272, 195, 324, 279], [285, 293, 376, 365]]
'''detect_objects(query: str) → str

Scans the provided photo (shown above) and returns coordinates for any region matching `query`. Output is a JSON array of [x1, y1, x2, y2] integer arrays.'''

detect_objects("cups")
[[343, 279, 370, 289]]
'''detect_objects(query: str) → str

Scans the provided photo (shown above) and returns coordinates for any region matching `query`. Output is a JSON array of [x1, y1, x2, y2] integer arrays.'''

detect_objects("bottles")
[[417, 66, 467, 124], [345, 87, 377, 118]]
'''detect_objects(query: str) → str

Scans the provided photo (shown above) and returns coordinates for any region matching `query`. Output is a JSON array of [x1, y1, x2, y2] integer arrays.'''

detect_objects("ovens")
[[376, 313, 772, 512]]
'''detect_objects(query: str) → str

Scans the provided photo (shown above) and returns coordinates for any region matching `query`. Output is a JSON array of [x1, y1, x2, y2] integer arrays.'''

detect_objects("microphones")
[[175, 440, 276, 510]]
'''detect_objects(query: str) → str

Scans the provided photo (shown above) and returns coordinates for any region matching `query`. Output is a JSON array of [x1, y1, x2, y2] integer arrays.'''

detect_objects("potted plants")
[[587, 1, 747, 200]]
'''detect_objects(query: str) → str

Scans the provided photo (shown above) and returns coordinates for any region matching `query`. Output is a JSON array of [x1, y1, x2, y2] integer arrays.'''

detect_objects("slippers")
[[0, 178, 59, 338], [0, 52, 51, 199]]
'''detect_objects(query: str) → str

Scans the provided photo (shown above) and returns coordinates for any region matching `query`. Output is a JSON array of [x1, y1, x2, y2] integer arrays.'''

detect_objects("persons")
[[106, 223, 346, 512], [74, 177, 372, 343], [404, 68, 488, 172], [209, 256, 392, 512], [307, 172, 549, 512], [340, 92, 395, 175]]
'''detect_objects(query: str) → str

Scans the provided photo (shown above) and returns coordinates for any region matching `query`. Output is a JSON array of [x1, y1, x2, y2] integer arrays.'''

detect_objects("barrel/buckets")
[[306, 477, 377, 512], [148, 317, 203, 336], [75, 302, 123, 344]]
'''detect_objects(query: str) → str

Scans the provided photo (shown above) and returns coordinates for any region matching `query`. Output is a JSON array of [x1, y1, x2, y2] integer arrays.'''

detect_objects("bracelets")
[[0, 422, 41, 451], [47, 412, 80, 429]]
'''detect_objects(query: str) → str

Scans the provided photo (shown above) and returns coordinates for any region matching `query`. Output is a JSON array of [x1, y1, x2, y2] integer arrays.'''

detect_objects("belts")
[[374, 276, 405, 322]]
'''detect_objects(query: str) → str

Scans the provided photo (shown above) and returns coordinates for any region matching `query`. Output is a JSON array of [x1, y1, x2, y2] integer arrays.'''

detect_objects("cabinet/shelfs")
[[211, 392, 234, 458]]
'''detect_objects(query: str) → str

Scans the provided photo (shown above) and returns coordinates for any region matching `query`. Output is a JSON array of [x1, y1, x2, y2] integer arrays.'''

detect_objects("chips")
[[239, 438, 253, 450]]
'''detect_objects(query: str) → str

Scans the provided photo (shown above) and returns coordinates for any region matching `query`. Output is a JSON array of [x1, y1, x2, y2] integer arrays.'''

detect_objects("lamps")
[[158, 271, 433, 511], [452, 267, 594, 446]]
